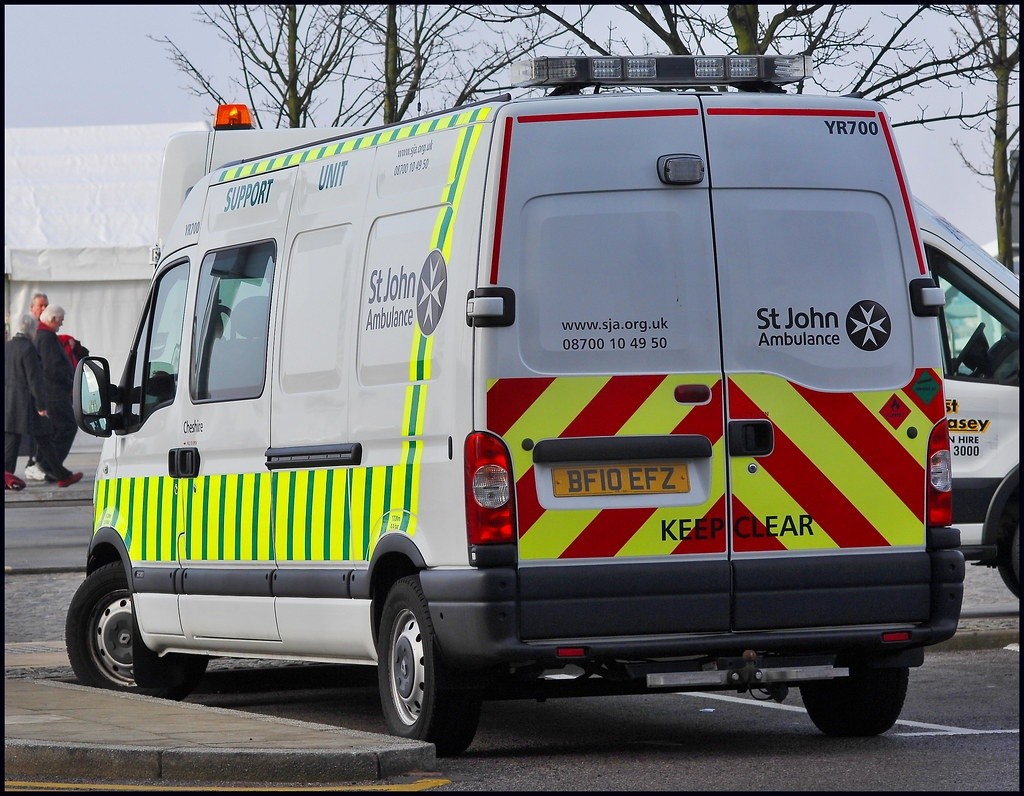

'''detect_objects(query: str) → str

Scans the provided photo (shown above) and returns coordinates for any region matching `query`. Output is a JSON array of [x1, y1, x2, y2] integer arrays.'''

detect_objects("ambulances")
[[911, 195, 1020, 603], [62, 50, 968, 758]]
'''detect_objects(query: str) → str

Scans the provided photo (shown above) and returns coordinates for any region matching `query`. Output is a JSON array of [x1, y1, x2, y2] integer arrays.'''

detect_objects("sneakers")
[[25, 465, 46, 481], [57, 472, 83, 487], [5, 472, 26, 490]]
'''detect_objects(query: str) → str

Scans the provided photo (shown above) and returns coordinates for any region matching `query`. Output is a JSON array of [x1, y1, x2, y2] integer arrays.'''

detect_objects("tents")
[[5, 120, 213, 413]]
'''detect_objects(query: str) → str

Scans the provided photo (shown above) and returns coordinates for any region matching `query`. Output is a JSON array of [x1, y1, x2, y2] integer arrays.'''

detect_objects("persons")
[[5, 293, 85, 491]]
